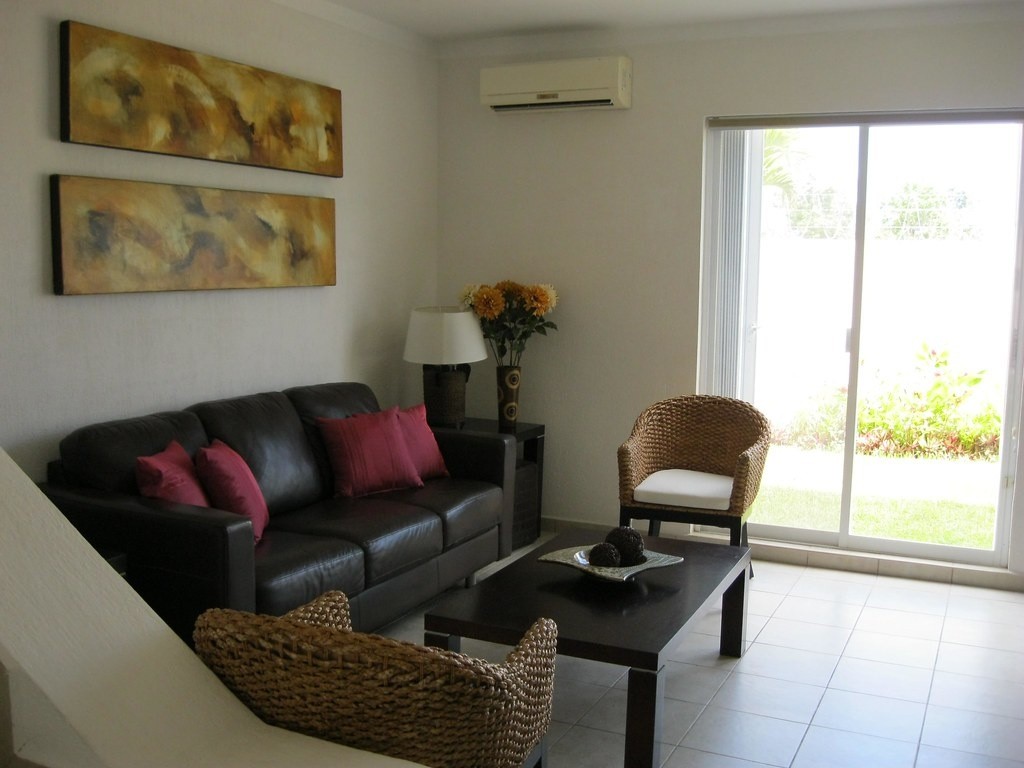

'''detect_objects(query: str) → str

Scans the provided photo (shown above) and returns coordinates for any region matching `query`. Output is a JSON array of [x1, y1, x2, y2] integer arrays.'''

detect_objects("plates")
[[538, 540, 685, 583]]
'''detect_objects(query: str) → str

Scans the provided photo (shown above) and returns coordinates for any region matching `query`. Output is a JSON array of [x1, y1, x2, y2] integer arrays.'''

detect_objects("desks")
[[423, 525, 751, 768]]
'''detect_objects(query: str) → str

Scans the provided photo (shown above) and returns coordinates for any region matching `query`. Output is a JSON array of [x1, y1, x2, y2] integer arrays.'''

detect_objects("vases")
[[495, 365, 521, 428]]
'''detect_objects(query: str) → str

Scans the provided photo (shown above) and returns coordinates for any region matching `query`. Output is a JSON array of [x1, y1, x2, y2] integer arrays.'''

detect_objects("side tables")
[[461, 416, 545, 550]]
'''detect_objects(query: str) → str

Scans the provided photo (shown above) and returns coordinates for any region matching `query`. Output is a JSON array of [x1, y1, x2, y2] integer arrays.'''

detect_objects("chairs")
[[194, 588, 559, 768], [616, 394, 771, 578]]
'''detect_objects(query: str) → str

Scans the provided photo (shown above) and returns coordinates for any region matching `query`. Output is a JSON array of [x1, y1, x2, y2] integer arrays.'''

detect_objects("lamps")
[[402, 305, 489, 424]]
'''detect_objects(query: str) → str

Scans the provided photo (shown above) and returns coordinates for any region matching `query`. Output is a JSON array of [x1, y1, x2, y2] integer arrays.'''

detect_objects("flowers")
[[457, 278, 558, 368]]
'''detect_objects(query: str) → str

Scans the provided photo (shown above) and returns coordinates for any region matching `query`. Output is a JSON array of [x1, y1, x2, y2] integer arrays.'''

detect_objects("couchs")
[[35, 382, 517, 662]]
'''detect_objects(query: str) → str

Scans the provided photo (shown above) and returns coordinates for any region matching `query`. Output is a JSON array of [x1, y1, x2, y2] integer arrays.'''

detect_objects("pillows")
[[315, 404, 425, 502], [350, 402, 449, 480], [195, 438, 270, 546], [137, 440, 210, 508]]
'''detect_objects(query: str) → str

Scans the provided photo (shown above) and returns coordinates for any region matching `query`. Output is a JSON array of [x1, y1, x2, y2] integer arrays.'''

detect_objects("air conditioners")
[[478, 55, 634, 115]]
